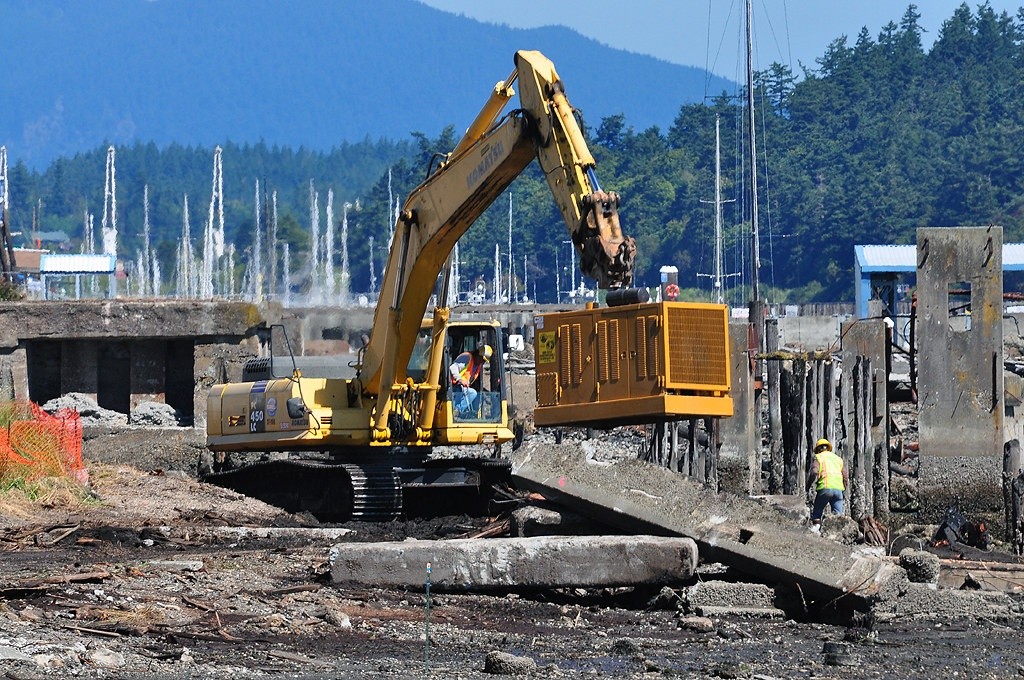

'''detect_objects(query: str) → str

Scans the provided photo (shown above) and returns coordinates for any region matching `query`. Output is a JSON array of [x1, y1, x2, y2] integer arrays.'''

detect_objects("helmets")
[[478, 345, 493, 362], [813, 438, 831, 450]]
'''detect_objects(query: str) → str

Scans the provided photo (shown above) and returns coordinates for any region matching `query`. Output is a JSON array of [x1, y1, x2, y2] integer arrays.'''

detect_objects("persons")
[[805, 439, 847, 528], [449, 345, 493, 420]]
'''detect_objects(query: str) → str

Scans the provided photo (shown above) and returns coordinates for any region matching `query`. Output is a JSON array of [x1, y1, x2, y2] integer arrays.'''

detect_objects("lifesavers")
[[665, 284, 681, 298]]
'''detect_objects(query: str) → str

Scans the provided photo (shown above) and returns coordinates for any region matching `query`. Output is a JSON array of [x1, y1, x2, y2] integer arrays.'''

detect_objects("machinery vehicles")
[[198, 51, 651, 526]]
